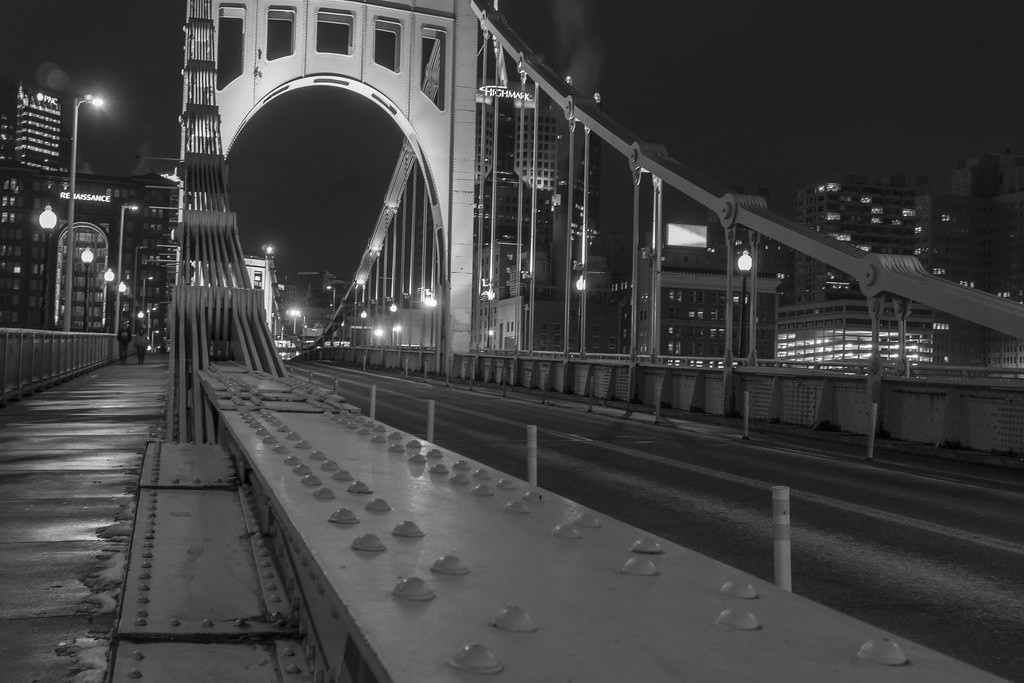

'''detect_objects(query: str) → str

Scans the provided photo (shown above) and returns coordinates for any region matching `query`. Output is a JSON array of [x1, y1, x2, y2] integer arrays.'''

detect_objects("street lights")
[[80, 245, 94, 332], [39, 200, 57, 330], [736, 247, 753, 356], [575, 270, 586, 352], [101, 265, 115, 333], [116, 279, 126, 333]]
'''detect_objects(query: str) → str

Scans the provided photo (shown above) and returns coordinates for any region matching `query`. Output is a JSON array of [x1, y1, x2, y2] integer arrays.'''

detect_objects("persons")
[[117, 322, 132, 363], [134, 329, 148, 364]]
